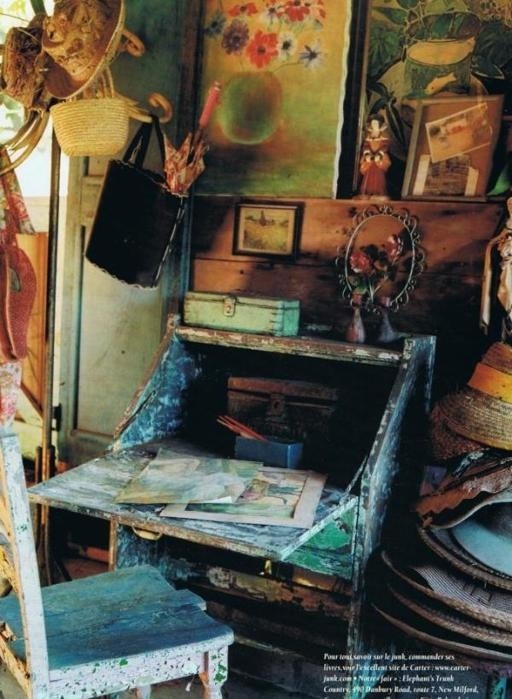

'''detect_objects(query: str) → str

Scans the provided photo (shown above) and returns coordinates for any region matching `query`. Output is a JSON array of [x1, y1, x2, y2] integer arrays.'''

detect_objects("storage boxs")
[[184, 291, 300, 337]]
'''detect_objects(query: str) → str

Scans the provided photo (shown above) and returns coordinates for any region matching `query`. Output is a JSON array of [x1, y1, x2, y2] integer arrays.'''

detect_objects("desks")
[[28, 314, 438, 698]]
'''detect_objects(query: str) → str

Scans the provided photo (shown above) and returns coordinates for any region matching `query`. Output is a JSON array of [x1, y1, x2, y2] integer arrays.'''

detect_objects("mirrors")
[[334, 203, 428, 346]]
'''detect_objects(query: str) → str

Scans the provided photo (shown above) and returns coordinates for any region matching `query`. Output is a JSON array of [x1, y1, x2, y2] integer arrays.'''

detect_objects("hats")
[[431, 341, 512, 451]]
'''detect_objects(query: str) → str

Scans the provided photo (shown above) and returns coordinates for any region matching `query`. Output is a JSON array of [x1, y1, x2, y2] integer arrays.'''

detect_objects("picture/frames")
[[232, 203, 302, 261], [401, 94, 504, 203]]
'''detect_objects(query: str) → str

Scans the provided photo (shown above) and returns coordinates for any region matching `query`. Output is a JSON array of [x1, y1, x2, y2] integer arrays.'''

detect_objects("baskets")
[[48, 98, 130, 157]]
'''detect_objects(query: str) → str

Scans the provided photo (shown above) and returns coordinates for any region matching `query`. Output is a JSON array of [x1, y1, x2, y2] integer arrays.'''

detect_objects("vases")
[[345, 295, 365, 343]]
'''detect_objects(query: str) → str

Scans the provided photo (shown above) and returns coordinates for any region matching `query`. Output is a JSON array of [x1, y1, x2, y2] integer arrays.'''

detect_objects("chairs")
[[1, 348, 239, 699]]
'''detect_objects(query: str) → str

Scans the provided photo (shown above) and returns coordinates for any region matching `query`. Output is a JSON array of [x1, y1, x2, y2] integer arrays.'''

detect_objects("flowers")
[[344, 251, 386, 303], [374, 230, 407, 299]]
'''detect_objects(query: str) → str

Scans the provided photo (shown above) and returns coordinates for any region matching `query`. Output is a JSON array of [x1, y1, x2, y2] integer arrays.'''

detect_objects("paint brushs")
[[216, 415, 268, 441]]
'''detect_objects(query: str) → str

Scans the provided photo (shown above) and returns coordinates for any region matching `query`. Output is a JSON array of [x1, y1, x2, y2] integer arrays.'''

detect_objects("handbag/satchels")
[[83, 113, 187, 292]]
